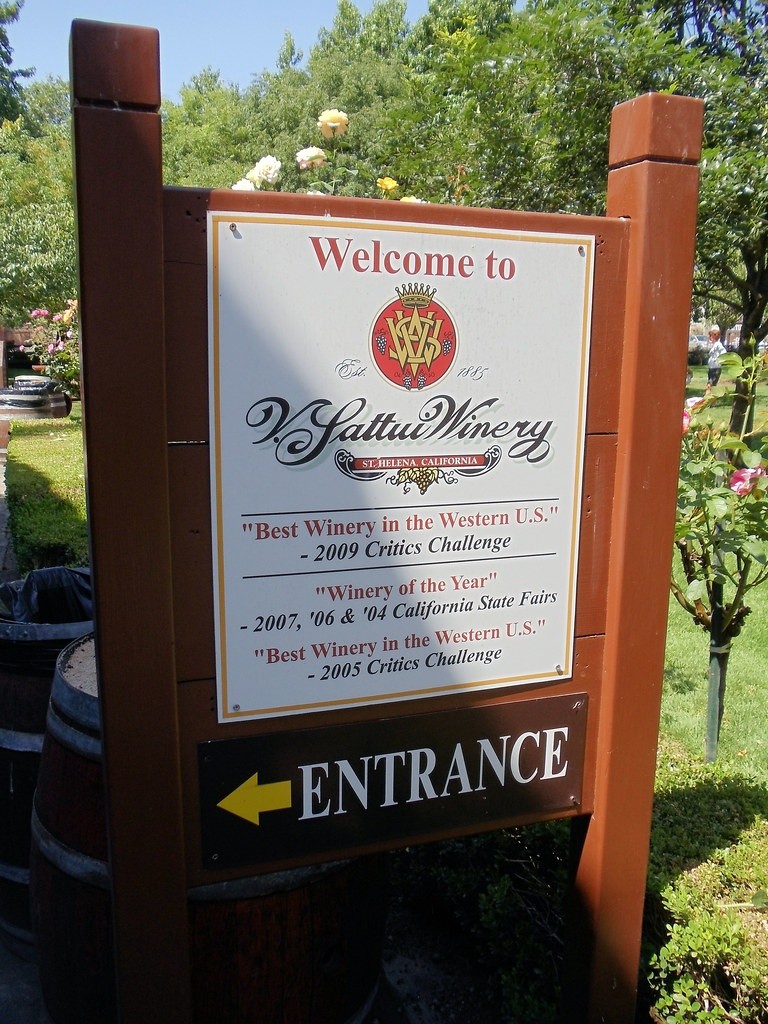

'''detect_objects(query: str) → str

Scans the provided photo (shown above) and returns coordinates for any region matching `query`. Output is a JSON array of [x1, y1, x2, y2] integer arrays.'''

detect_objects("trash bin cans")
[[0, 566, 91, 966], [31, 632, 116, 1024]]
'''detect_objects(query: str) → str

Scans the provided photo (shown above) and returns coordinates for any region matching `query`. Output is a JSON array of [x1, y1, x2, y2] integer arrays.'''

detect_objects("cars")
[[688, 323, 768, 352]]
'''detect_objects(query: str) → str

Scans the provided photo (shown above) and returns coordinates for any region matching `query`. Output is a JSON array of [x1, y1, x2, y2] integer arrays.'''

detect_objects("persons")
[[705, 330, 727, 407]]
